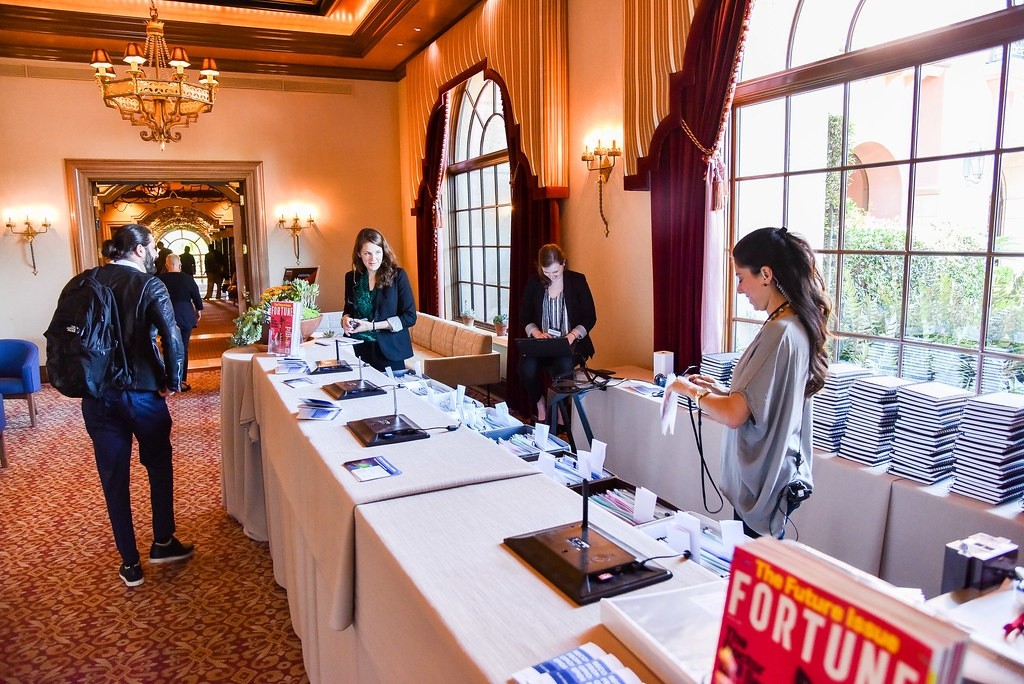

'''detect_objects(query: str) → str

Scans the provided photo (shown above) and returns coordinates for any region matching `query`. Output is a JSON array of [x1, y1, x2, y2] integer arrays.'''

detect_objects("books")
[[674, 350, 1024, 506]]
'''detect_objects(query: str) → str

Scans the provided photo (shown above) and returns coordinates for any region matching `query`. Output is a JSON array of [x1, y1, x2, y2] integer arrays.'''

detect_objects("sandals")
[[181, 383, 190, 392]]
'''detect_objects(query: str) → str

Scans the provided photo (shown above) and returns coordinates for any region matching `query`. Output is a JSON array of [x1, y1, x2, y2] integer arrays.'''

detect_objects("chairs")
[[0, 338, 40, 426], [0, 393, 7, 468]]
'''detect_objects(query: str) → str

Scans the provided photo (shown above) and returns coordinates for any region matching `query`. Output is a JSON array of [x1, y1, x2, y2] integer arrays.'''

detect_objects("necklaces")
[[769, 303, 791, 321]]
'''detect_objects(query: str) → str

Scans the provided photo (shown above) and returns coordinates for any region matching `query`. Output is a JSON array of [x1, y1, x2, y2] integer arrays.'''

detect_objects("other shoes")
[[204, 296, 211, 300]]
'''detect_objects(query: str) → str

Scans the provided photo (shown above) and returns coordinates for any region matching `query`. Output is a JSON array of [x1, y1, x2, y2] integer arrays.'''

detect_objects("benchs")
[[402, 310, 500, 407]]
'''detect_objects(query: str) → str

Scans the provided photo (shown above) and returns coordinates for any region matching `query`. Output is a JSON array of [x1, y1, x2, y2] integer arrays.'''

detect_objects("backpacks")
[[46, 266, 122, 398]]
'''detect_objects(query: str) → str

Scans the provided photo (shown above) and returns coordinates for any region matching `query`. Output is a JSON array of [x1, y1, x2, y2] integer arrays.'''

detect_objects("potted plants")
[[462, 307, 475, 327], [493, 314, 508, 336]]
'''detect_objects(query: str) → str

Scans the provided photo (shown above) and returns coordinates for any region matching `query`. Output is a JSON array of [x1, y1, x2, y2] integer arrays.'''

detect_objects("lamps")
[[279, 211, 314, 265], [90, 0, 220, 153], [581, 139, 622, 237], [5, 208, 51, 275]]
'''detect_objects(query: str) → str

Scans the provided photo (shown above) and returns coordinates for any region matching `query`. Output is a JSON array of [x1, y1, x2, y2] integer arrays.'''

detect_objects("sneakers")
[[119, 561, 145, 586], [149, 537, 194, 564]]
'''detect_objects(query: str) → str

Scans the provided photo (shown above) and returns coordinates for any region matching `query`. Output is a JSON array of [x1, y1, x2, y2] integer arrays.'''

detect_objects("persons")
[[156, 254, 203, 392], [340, 228, 416, 372], [155, 241, 173, 272], [204, 244, 224, 299], [179, 246, 197, 278], [667, 227, 844, 540], [515, 244, 597, 433], [57, 225, 194, 587]]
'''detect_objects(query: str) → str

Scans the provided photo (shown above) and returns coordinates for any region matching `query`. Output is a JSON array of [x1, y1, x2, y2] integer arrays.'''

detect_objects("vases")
[[262, 314, 324, 342]]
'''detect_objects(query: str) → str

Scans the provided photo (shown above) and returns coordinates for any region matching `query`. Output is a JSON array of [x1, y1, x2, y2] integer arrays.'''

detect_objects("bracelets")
[[570, 332, 576, 339], [373, 322, 375, 331]]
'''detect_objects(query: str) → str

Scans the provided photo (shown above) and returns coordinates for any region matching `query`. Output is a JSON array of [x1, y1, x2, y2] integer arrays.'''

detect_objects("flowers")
[[227, 278, 322, 348]]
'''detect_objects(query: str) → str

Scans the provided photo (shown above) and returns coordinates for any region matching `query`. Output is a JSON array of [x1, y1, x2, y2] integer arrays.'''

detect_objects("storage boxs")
[[382, 369, 430, 384], [570, 477, 684, 527], [455, 406, 524, 431], [404, 378, 455, 398], [637, 510, 754, 578], [483, 424, 571, 458], [427, 393, 485, 413], [523, 450, 617, 487]]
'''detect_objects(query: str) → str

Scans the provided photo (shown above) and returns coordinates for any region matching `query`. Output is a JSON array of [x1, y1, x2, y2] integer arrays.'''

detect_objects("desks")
[[220, 340, 1024, 684]]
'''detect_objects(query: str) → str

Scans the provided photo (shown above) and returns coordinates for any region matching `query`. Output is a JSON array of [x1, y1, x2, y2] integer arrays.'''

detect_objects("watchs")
[[695, 387, 712, 409]]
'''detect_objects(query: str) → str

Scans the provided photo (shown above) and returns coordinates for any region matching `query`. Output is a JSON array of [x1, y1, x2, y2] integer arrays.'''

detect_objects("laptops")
[[515, 338, 574, 358]]
